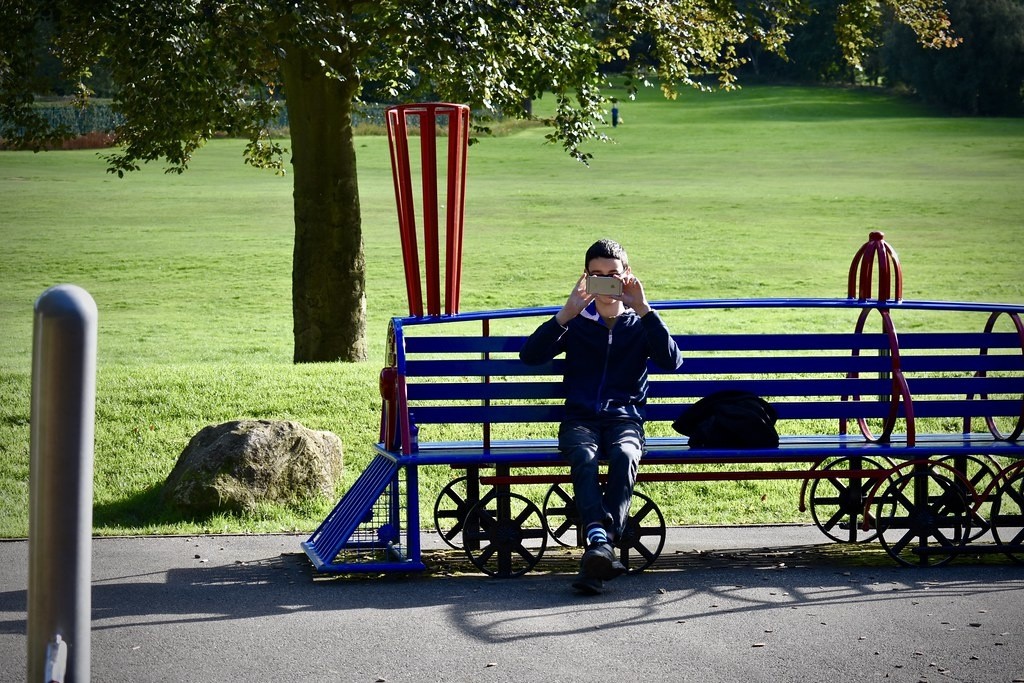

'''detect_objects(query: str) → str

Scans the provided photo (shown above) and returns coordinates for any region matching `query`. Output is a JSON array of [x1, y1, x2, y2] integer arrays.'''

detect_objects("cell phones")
[[586, 276, 623, 296]]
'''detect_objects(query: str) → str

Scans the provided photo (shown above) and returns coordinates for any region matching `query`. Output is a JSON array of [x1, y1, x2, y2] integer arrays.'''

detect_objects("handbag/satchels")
[[670, 388, 781, 453]]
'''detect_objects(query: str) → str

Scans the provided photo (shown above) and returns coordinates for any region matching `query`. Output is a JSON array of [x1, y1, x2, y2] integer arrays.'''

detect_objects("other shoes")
[[571, 573, 604, 594], [582, 540, 627, 581]]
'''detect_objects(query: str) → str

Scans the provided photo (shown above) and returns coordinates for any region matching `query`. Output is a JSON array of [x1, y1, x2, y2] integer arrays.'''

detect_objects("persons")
[[519, 239, 685, 597]]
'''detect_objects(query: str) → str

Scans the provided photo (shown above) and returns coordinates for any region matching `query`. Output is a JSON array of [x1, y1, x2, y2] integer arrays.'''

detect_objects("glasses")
[[586, 268, 627, 277]]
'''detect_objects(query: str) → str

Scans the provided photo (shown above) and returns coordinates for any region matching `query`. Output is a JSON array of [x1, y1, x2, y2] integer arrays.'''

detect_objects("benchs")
[[300, 102, 1024, 577]]
